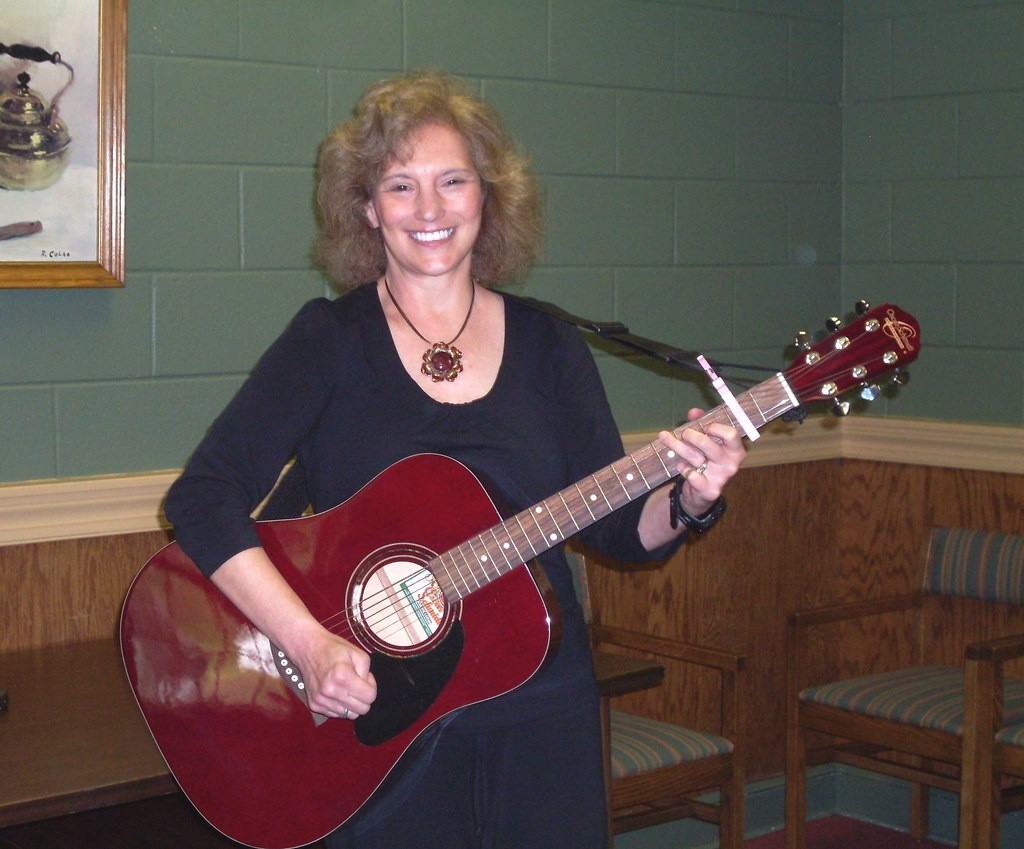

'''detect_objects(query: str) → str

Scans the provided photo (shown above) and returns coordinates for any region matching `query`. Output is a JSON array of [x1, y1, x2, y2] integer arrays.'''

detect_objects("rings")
[[696, 461, 707, 473], [339, 709, 348, 718]]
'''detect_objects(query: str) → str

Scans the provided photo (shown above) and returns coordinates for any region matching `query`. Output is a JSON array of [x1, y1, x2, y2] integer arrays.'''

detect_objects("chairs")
[[782, 525, 1024, 849], [562, 546, 748, 848]]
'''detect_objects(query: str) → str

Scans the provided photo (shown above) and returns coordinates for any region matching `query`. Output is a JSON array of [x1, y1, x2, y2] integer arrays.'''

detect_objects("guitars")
[[115, 283, 919, 849]]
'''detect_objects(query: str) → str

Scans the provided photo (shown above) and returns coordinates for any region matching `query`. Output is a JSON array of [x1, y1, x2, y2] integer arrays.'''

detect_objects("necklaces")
[[385, 277, 474, 383]]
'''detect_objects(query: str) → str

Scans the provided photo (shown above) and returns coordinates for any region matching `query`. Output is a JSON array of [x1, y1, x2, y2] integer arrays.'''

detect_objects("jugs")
[[0, 41, 75, 192]]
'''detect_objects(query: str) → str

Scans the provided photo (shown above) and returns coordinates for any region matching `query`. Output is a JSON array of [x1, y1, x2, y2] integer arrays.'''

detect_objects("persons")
[[160, 79, 747, 849]]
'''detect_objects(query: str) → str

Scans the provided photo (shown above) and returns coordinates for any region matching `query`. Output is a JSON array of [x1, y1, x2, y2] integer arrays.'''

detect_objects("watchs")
[[669, 477, 726, 535]]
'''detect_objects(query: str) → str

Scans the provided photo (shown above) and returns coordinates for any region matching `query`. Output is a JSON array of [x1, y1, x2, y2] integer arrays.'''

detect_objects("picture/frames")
[[0, 1, 128, 288]]
